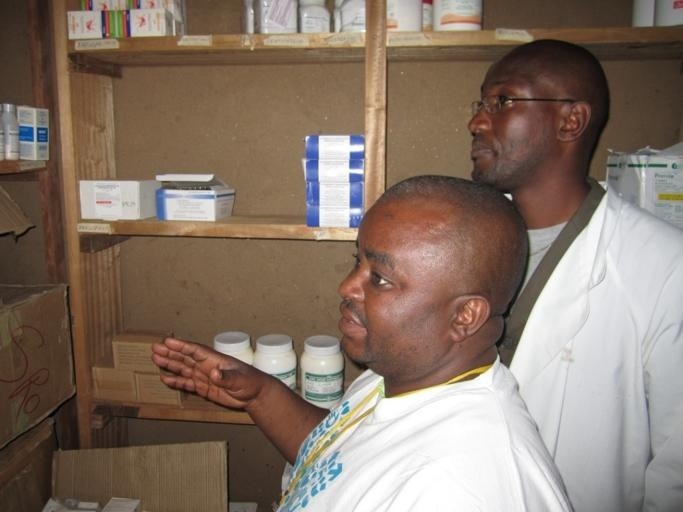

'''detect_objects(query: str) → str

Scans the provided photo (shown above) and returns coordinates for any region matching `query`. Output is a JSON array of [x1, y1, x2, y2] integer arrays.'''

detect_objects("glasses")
[[472, 93, 577, 115]]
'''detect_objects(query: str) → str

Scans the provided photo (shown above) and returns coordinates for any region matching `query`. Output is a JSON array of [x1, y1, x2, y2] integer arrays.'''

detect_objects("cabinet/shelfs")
[[0, 0, 681, 510]]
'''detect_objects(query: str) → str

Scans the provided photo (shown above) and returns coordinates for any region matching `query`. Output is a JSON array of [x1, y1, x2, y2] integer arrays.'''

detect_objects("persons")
[[469, 39, 683, 512], [152, 175, 575, 512]]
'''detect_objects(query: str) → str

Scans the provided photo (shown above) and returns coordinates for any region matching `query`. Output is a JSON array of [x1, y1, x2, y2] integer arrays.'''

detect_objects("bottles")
[[242, 0, 485, 33], [212, 331, 344, 411], [633, 0, 683, 29], [0, 101, 20, 162]]
